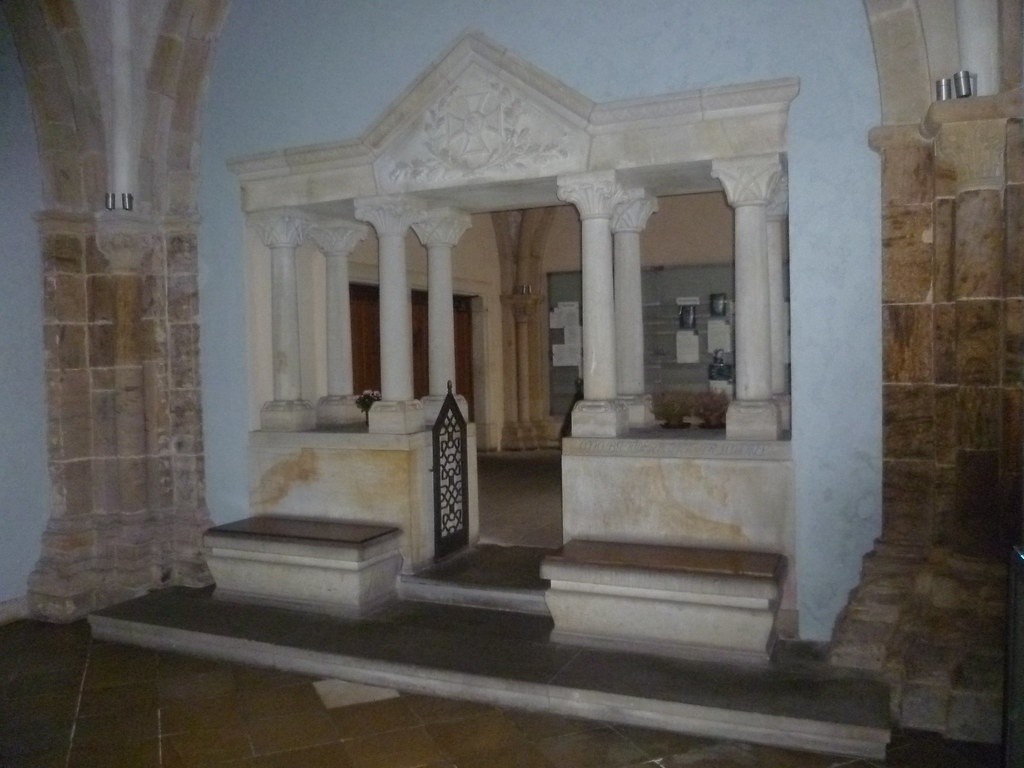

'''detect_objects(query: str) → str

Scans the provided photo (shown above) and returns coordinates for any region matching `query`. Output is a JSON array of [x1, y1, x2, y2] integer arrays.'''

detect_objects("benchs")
[[199, 512, 405, 623], [538, 538, 789, 667]]
